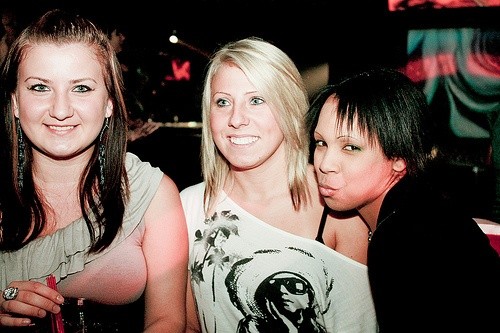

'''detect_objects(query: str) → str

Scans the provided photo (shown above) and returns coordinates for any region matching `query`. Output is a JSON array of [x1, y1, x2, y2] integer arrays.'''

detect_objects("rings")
[[3, 287, 19, 302]]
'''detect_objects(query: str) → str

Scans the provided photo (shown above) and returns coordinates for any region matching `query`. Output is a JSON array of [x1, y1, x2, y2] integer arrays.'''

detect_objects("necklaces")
[[368, 211, 395, 241]]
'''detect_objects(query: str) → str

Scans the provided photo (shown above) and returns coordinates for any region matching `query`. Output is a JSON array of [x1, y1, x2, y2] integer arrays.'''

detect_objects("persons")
[[178, 37, 380, 333], [305, 68, 500, 333], [0, 8, 189, 333]]
